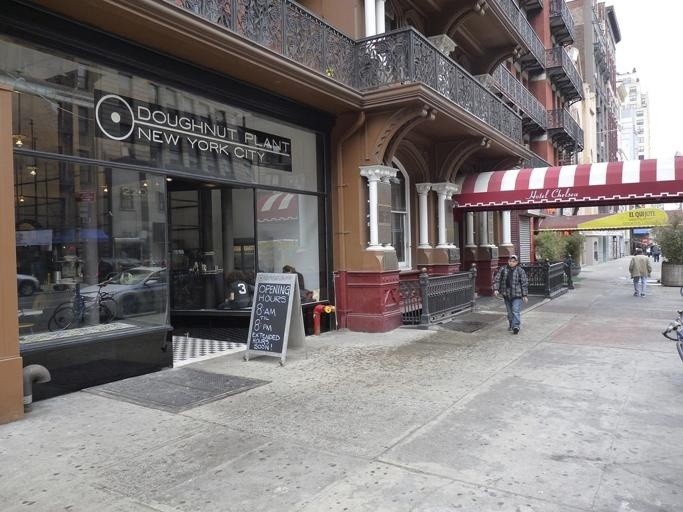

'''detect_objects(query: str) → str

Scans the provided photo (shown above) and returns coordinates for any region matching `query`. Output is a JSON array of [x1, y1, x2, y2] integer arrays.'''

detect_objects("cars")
[[17, 274, 39, 296], [71, 265, 167, 318]]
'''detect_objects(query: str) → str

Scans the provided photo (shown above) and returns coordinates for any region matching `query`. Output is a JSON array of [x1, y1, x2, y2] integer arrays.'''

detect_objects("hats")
[[509, 255, 518, 260]]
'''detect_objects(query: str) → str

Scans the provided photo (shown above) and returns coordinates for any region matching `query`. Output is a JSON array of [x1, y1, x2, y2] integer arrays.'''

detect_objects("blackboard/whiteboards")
[[247, 273, 296, 358]]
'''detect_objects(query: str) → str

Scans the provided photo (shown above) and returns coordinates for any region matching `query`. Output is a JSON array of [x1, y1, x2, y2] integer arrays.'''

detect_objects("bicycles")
[[48, 283, 119, 331], [663, 310, 683, 361]]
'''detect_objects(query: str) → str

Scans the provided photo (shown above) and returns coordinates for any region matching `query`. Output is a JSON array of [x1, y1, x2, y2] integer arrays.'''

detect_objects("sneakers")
[[633, 292, 646, 297], [508, 327, 520, 335]]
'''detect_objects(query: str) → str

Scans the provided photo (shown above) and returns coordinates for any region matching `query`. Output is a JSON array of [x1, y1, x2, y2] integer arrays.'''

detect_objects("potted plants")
[[650, 211, 683, 287], [560, 233, 584, 276]]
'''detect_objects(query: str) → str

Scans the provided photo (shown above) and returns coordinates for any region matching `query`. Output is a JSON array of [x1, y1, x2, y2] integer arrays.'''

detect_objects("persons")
[[629, 249, 652, 297], [493, 255, 529, 334], [631, 241, 661, 262], [218, 270, 255, 311], [282, 265, 304, 292], [561, 252, 574, 290]]
